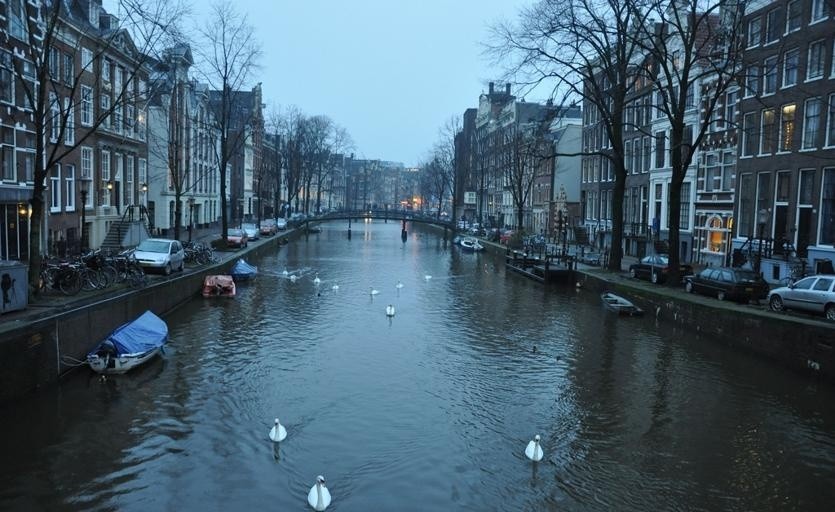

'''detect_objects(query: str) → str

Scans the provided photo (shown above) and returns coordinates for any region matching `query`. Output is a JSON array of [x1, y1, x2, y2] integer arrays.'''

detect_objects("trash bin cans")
[[0, 260, 29, 314]]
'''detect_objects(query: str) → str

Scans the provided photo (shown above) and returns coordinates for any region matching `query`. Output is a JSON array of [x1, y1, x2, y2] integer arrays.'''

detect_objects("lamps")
[[141, 183, 148, 198], [104, 181, 113, 196]]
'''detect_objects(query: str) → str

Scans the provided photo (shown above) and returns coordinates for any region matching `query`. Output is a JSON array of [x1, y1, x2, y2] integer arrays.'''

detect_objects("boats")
[[229, 259, 260, 283], [84, 309, 172, 377], [598, 290, 648, 319], [201, 275, 238, 301], [453, 235, 486, 252], [308, 226, 325, 234]]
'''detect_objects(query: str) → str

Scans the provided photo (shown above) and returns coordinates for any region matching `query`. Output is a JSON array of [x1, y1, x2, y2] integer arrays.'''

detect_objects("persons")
[[211, 283, 224, 296]]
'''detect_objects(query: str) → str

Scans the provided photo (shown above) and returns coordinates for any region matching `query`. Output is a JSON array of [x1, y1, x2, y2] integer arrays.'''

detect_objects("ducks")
[[526, 346, 538, 353], [548, 355, 563, 364]]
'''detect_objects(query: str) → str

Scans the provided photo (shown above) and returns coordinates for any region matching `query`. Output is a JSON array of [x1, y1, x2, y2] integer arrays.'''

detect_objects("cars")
[[681, 265, 771, 305], [765, 274, 835, 323], [239, 223, 261, 242], [258, 213, 306, 238], [128, 237, 187, 277], [628, 254, 696, 287], [313, 206, 331, 217], [487, 227, 602, 266], [227, 228, 249, 250], [438, 214, 488, 236]]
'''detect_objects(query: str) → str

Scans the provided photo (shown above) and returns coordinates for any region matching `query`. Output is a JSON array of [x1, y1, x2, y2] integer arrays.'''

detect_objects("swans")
[[385, 304, 395, 316], [525, 434, 544, 463], [368, 286, 380, 295], [396, 279, 404, 289], [332, 279, 339, 290], [307, 474, 332, 511], [313, 271, 321, 284], [283, 264, 288, 275], [424, 271, 433, 280], [268, 417, 288, 443]]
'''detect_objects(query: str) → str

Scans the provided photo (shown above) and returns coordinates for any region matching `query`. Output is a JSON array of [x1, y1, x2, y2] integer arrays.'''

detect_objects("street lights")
[[562, 206, 570, 259], [76, 174, 93, 253], [497, 207, 502, 245], [187, 189, 198, 242], [756, 207, 771, 276], [463, 205, 467, 232], [239, 197, 246, 230]]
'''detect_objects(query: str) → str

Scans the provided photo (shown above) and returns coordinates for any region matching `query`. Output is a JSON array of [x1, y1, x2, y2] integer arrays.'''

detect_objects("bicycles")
[[180, 239, 224, 270], [33, 246, 152, 298]]
